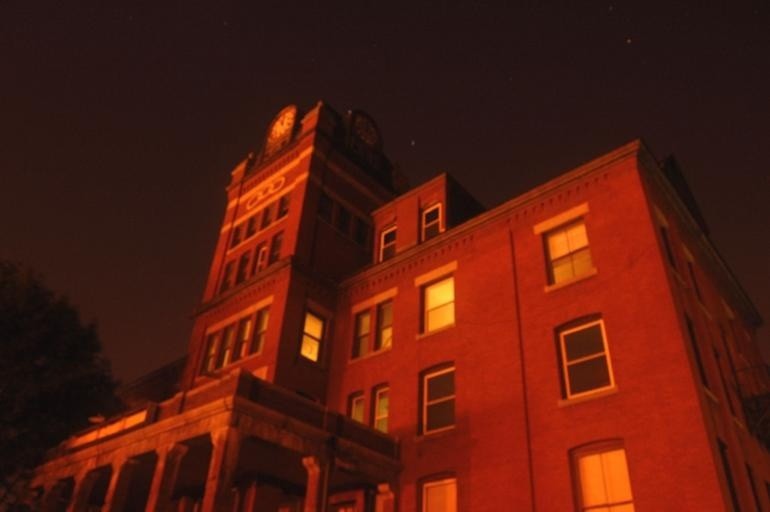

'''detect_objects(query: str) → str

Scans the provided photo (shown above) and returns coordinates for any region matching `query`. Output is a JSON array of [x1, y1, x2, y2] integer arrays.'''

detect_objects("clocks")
[[267, 106, 294, 142], [354, 113, 377, 145]]
